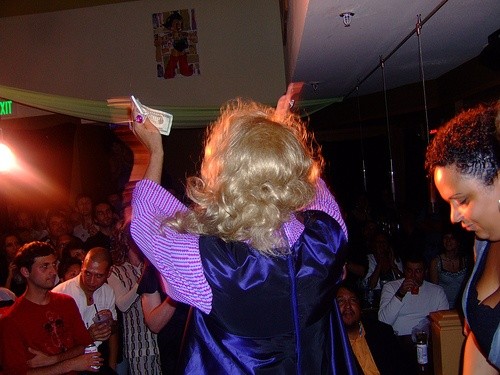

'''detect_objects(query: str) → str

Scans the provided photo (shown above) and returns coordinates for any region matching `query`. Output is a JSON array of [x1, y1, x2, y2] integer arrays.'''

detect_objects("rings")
[[135, 114, 144, 124]]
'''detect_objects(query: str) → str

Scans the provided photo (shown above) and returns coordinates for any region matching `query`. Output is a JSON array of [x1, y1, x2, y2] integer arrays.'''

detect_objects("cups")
[[92, 314, 111, 340], [410, 283, 419, 295]]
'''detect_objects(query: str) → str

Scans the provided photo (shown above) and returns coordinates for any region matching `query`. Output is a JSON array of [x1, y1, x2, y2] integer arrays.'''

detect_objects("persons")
[[377, 255, 450, 375], [130, 81, 383, 375], [0, 187, 196, 307], [112, 219, 162, 375], [425, 100, 500, 374], [334, 281, 400, 375], [50, 247, 117, 375], [137, 258, 196, 375], [337, 196, 477, 311], [0, 242, 104, 375]]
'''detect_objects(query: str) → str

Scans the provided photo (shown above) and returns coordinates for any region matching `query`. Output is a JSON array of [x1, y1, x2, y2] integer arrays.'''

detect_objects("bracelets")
[[397, 289, 405, 297]]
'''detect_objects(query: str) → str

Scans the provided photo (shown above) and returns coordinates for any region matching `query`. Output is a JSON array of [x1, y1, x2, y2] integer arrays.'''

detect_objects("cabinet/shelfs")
[[430, 311, 464, 375]]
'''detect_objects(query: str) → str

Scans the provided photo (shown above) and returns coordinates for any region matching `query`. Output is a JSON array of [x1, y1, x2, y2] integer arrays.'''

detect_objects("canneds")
[[411, 280, 418, 294], [84, 344, 100, 369]]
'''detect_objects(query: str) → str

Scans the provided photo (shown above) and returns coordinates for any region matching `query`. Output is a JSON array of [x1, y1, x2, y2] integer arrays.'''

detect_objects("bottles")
[[85, 343, 100, 370], [416, 319, 429, 365]]
